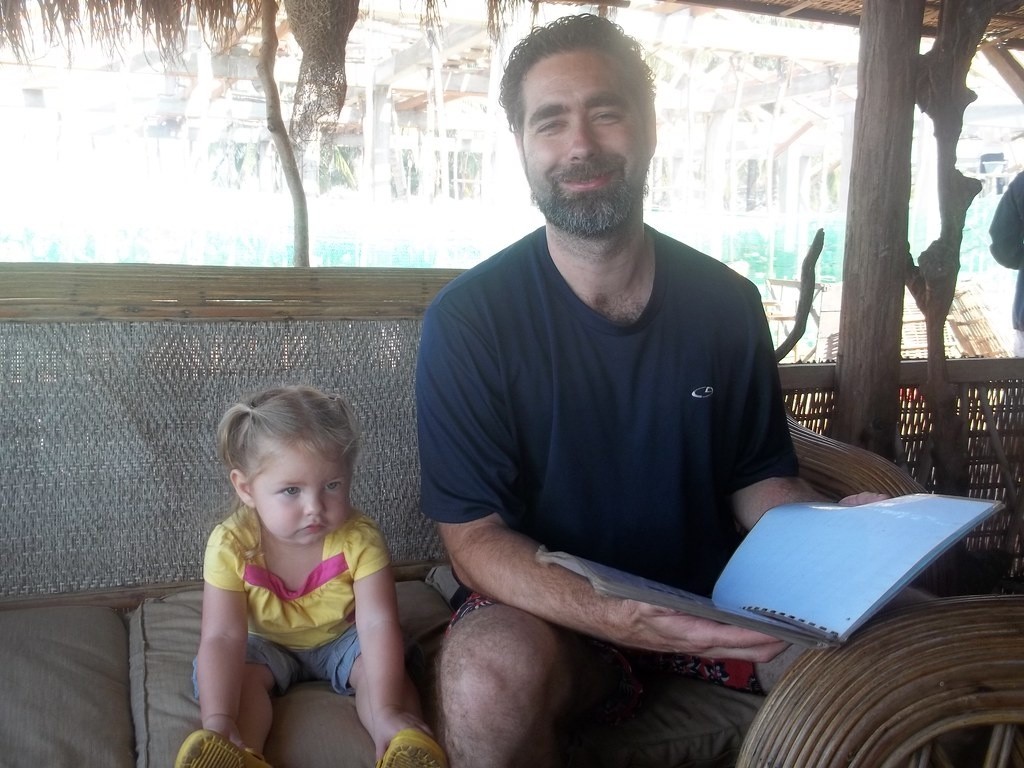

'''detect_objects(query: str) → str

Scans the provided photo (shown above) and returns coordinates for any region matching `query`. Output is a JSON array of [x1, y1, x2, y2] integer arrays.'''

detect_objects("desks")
[[735, 595, 1024, 768]]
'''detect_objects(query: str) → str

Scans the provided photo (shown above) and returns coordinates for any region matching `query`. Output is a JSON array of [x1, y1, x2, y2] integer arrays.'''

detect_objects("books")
[[536, 493, 1005, 648]]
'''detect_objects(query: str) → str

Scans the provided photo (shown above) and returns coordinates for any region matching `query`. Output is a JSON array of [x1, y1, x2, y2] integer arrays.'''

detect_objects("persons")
[[420, 13, 992, 767], [989, 172, 1024, 357], [174, 384, 450, 767]]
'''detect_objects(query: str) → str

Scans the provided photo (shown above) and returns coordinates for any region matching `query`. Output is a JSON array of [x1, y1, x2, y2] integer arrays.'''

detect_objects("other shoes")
[[374, 729, 447, 768], [174, 730, 273, 767]]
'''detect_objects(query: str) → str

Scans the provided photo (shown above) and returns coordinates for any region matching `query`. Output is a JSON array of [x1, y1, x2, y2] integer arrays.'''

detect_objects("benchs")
[[0, 260, 1023, 768]]
